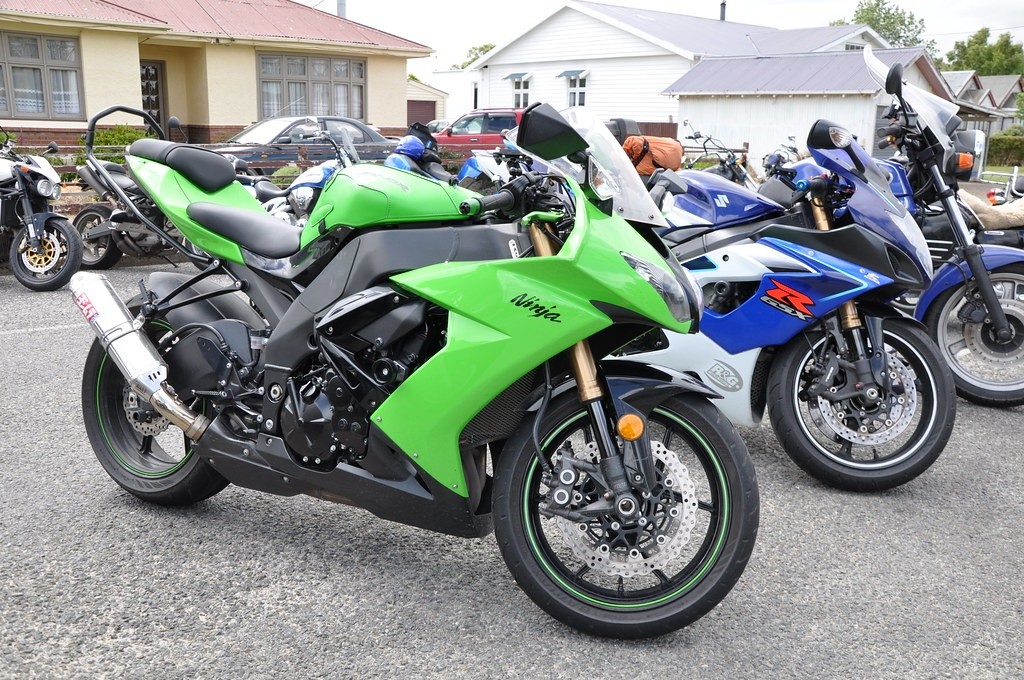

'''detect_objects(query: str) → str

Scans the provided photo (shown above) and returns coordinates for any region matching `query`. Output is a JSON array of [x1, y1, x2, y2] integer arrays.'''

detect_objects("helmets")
[[394, 134, 426, 161]]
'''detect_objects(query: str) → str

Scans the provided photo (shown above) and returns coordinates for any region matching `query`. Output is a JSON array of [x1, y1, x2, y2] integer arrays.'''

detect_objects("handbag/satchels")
[[621, 136, 683, 176]]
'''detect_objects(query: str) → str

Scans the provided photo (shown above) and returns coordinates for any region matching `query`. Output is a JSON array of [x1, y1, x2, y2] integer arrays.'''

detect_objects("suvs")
[[432, 107, 526, 172]]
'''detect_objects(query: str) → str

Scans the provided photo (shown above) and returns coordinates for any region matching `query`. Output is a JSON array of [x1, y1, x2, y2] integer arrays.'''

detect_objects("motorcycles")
[[884, 163, 1023, 316], [74, 86, 756, 279], [67, 104, 760, 640], [457, 119, 960, 494], [749, 46, 1024, 411], [0, 129, 84, 290]]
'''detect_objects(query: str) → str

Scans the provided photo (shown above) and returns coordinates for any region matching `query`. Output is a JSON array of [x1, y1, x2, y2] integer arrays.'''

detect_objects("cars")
[[183, 114, 406, 174], [424, 118, 482, 137]]
